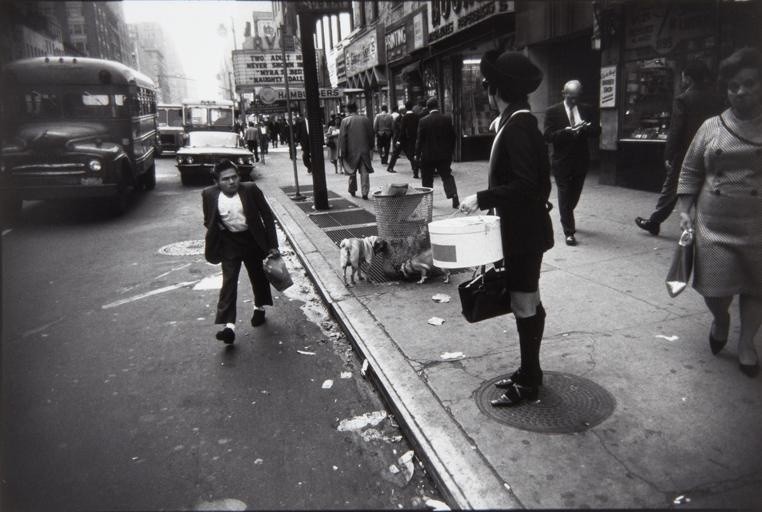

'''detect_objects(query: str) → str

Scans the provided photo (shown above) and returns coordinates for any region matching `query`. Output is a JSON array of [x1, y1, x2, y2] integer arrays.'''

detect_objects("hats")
[[480, 49, 543, 96]]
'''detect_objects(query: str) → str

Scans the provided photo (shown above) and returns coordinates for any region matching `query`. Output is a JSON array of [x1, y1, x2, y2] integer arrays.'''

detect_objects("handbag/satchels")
[[262, 254, 293, 291], [665, 229, 694, 298], [458, 267, 513, 322]]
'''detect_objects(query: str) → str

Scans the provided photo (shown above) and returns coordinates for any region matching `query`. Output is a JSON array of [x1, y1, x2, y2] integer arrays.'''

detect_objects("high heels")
[[491, 381, 538, 406], [495, 368, 543, 387]]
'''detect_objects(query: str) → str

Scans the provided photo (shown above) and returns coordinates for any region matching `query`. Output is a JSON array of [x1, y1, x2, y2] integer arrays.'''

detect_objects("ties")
[[569, 106, 576, 129]]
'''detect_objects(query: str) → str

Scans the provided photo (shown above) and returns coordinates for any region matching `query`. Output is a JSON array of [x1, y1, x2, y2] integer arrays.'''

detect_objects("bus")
[[2, 56, 161, 221]]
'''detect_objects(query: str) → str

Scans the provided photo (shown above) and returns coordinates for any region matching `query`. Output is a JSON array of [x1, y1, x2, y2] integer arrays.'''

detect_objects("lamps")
[[589, 30, 621, 54]]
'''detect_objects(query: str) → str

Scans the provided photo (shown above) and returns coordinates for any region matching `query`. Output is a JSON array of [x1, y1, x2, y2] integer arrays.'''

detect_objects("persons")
[[675, 45, 761, 377], [240, 95, 460, 210], [544, 80, 601, 245], [635, 59, 718, 234], [461, 50, 555, 408], [200, 159, 279, 343]]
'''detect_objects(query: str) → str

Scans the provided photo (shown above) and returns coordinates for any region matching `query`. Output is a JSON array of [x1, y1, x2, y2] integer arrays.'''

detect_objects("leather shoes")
[[566, 235, 574, 245], [216, 327, 235, 344], [739, 360, 759, 378], [636, 216, 659, 235], [251, 309, 264, 325], [710, 313, 730, 354]]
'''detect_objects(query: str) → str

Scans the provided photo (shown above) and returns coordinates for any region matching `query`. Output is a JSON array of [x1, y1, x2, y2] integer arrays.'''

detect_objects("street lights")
[[217, 14, 238, 52]]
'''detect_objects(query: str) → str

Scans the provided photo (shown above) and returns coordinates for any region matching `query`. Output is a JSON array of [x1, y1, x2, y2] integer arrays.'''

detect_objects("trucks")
[[177, 100, 239, 145], [153, 104, 185, 157]]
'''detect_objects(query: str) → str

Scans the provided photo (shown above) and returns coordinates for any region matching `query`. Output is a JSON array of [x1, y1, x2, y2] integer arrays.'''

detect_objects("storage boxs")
[[428, 214, 504, 271]]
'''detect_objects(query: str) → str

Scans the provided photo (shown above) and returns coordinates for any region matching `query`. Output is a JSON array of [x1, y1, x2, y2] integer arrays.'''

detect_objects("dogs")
[[394, 248, 452, 284], [339, 235, 388, 286]]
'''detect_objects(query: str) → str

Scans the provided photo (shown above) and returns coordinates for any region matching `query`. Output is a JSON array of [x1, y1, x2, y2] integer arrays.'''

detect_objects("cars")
[[176, 130, 253, 186]]
